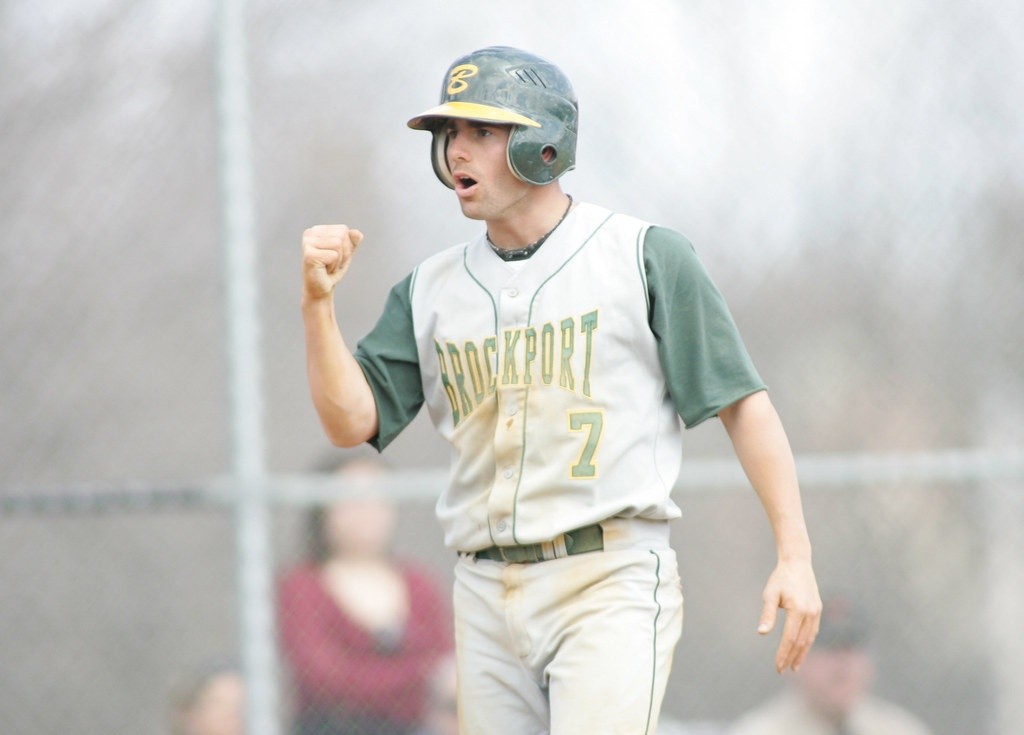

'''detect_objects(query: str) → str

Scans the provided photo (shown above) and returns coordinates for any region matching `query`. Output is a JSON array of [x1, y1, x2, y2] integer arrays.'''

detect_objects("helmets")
[[406, 48, 578, 188]]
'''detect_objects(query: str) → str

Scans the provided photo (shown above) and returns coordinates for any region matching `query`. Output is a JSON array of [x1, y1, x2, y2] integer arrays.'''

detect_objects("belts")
[[458, 524, 605, 564]]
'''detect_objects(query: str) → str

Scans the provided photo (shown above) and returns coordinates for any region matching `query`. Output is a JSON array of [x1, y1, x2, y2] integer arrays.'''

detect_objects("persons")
[[729, 592, 932, 735], [303, 45, 821, 735], [175, 452, 456, 735]]
